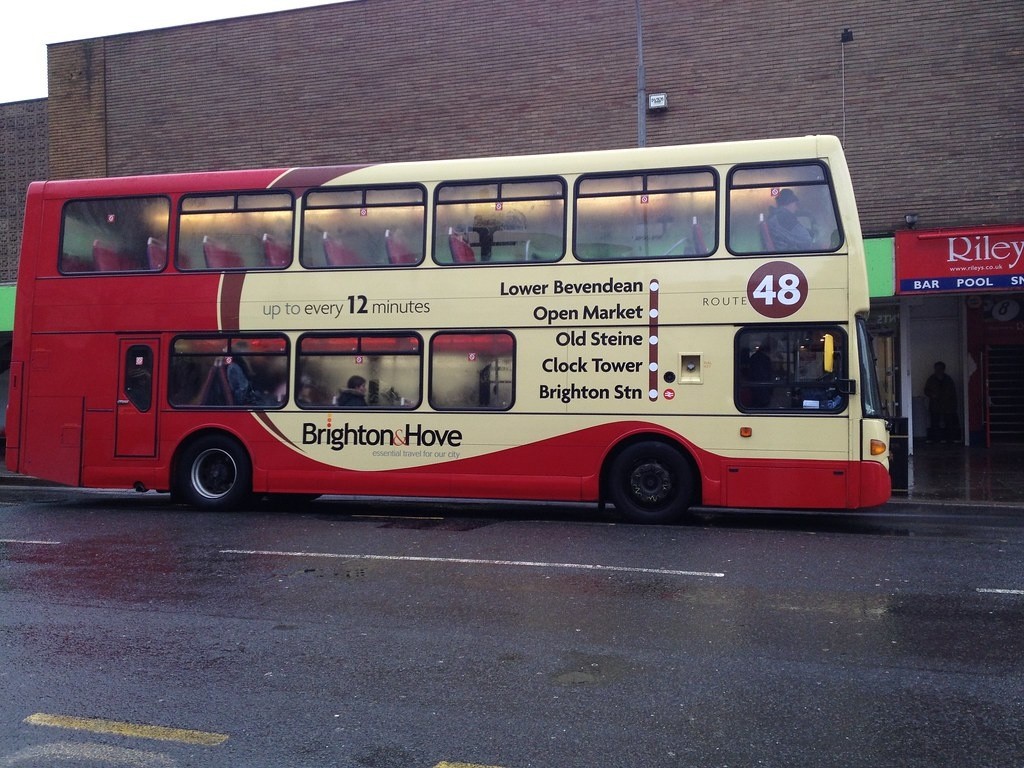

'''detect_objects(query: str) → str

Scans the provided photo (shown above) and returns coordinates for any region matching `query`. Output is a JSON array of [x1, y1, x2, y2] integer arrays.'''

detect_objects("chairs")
[[203, 237, 248, 267], [323, 231, 372, 266], [93, 239, 194, 271], [760, 213, 777, 252], [449, 227, 477, 263], [693, 216, 709, 254], [184, 356, 235, 405], [385, 230, 415, 264], [261, 233, 290, 267]]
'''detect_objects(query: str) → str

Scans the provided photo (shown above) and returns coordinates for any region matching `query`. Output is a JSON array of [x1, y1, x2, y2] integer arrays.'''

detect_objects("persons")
[[242, 225, 273, 267], [924, 361, 961, 444], [740, 337, 779, 407], [299, 373, 328, 405], [337, 375, 369, 405], [767, 188, 817, 250], [381, 387, 410, 403], [226, 341, 275, 405]]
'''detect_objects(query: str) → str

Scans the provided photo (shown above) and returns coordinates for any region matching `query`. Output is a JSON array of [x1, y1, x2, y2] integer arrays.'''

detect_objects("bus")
[[4, 132, 895, 525]]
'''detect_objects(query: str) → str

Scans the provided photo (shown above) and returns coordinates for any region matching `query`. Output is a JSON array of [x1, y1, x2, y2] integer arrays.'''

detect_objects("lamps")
[[904, 213, 919, 227]]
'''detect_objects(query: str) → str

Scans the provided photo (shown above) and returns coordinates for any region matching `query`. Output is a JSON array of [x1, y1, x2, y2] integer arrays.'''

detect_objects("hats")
[[774, 188, 798, 207]]
